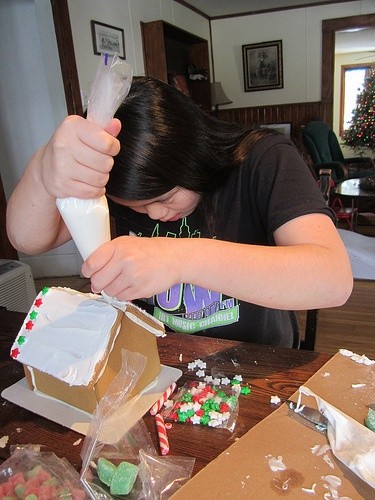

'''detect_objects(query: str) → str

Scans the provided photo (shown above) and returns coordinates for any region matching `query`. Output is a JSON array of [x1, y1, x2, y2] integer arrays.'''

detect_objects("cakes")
[[10, 287, 168, 422]]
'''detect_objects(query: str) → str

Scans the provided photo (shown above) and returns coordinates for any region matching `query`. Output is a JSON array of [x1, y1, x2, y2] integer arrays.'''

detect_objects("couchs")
[[301, 120, 375, 184]]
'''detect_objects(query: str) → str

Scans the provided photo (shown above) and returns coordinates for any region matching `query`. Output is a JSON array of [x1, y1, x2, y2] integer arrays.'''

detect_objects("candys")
[[0, 465, 87, 500], [365, 408, 375, 432], [150, 358, 251, 456], [270, 396, 281, 405], [97, 458, 140, 495]]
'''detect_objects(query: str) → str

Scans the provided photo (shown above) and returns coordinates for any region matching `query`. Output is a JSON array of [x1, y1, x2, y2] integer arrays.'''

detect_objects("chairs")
[[316, 178, 357, 233]]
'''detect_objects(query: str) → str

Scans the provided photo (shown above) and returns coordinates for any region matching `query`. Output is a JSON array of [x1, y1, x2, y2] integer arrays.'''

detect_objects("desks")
[[0, 309, 375, 500], [336, 178, 375, 237]]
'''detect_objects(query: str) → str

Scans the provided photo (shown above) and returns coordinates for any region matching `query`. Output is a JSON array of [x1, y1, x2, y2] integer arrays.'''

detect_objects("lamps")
[[211, 81, 233, 120]]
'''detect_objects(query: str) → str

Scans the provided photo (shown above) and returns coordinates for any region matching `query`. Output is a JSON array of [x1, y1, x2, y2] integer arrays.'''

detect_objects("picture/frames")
[[91, 19, 126, 61], [242, 39, 284, 93], [260, 122, 293, 142]]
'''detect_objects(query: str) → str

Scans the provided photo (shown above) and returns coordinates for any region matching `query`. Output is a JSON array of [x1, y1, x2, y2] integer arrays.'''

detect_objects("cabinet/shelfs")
[[140, 19, 212, 117]]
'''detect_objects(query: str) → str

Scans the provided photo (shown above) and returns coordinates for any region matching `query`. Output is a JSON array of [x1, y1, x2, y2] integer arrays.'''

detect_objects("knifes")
[[284, 399, 327, 428]]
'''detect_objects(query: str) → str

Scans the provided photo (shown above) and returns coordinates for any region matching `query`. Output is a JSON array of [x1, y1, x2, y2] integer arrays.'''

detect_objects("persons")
[[5, 74, 355, 349]]
